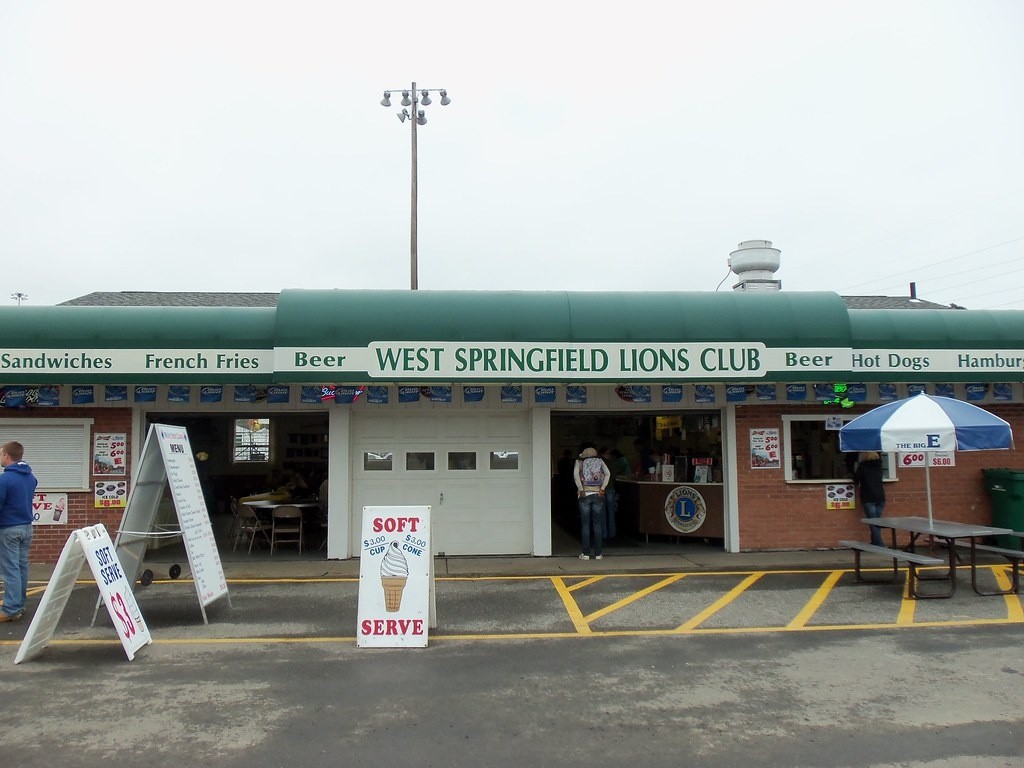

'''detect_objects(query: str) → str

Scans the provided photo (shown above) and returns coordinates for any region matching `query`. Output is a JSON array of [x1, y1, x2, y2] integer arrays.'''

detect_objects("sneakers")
[[578, 553, 589, 561], [594, 554, 603, 560]]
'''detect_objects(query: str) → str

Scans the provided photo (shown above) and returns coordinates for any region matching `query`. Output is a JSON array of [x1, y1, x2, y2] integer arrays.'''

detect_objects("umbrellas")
[[838, 389, 1017, 529]]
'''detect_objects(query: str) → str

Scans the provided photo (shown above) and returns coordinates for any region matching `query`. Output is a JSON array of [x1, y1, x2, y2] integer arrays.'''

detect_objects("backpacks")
[[581, 458, 605, 486]]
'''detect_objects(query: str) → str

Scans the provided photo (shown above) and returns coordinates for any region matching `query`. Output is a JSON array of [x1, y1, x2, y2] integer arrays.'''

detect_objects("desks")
[[239, 493, 323, 549], [860, 515, 1014, 600]]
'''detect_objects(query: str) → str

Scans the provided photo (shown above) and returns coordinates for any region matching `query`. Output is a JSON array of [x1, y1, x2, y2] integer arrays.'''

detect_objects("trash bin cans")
[[980, 466, 1024, 551]]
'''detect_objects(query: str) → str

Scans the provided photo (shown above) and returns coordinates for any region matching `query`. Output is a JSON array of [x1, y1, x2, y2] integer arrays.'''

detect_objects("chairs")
[[269, 505, 307, 556], [232, 504, 273, 555], [319, 523, 328, 552], [227, 495, 256, 544]]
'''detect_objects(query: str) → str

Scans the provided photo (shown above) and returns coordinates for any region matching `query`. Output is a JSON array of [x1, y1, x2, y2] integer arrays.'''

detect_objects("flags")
[[1, 383, 1013, 407]]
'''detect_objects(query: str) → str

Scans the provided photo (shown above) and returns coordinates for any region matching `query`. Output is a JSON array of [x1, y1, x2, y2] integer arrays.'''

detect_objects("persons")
[[853, 450, 888, 549], [610, 447, 633, 478], [631, 438, 657, 478], [701, 443, 722, 464], [1, 440, 39, 621], [598, 445, 617, 545], [573, 441, 612, 561]]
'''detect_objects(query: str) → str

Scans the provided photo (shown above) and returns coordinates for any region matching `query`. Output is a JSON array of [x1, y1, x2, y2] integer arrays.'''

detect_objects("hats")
[[578, 448, 597, 458]]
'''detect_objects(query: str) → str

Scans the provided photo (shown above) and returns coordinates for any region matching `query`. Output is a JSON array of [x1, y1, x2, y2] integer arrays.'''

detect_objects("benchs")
[[837, 539, 945, 601], [923, 537, 1024, 596]]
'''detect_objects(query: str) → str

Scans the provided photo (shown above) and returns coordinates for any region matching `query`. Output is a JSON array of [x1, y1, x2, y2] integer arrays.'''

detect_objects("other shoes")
[[0, 610, 22, 623]]
